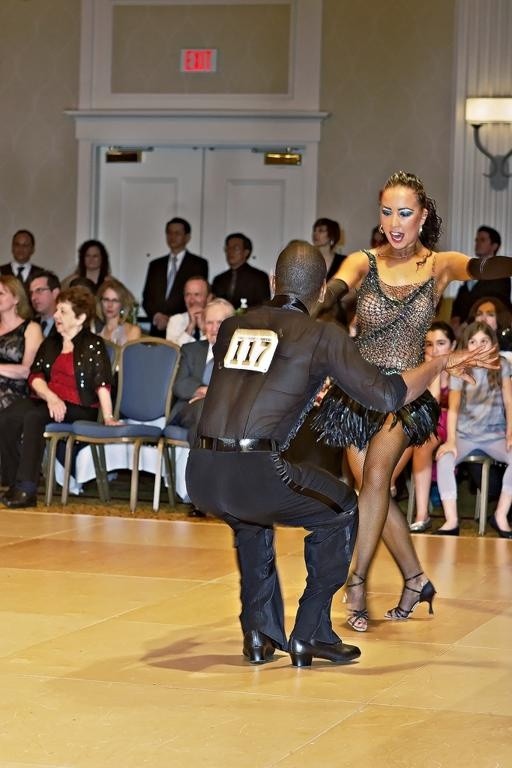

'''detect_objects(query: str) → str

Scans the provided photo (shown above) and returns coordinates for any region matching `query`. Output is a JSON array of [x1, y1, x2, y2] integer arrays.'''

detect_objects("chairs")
[[31, 336, 120, 504], [59, 335, 181, 515]]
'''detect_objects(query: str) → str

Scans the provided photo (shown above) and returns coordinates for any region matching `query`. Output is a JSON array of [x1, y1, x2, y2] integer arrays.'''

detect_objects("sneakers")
[[9, 487, 38, 508], [409, 517, 432, 532]]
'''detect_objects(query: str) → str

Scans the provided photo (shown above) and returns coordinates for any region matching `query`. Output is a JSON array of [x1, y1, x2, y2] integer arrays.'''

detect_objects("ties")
[[202, 357, 215, 385], [193, 325, 200, 341], [16, 267, 24, 282], [165, 257, 176, 300], [229, 271, 236, 296], [41, 321, 47, 332]]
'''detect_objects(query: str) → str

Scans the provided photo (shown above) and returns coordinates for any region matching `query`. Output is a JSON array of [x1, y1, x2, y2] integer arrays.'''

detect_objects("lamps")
[[463, 94, 511, 190]]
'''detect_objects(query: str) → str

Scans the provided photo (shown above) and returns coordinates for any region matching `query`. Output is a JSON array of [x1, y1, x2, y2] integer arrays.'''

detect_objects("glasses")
[[101, 296, 119, 303], [29, 287, 50, 295]]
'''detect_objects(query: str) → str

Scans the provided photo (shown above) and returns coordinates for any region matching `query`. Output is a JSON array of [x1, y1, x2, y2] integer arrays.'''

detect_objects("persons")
[[312, 171, 512, 633], [1, 214, 511, 540], [183, 238, 503, 670]]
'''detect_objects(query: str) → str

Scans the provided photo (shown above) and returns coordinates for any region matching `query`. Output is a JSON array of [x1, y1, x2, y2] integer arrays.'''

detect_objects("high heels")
[[384, 572, 435, 620], [343, 572, 369, 632]]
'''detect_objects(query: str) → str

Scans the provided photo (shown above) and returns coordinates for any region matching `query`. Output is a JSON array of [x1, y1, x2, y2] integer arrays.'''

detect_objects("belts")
[[193, 434, 280, 452]]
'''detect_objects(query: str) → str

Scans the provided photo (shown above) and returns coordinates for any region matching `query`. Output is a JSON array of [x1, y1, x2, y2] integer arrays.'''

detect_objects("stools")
[[150, 423, 190, 511], [404, 449, 493, 536]]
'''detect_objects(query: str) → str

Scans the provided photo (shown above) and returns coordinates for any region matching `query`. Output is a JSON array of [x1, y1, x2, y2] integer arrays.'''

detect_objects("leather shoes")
[[489, 511, 512, 537], [288, 638, 360, 666], [189, 503, 206, 516], [431, 526, 459, 535], [242, 630, 275, 664]]
